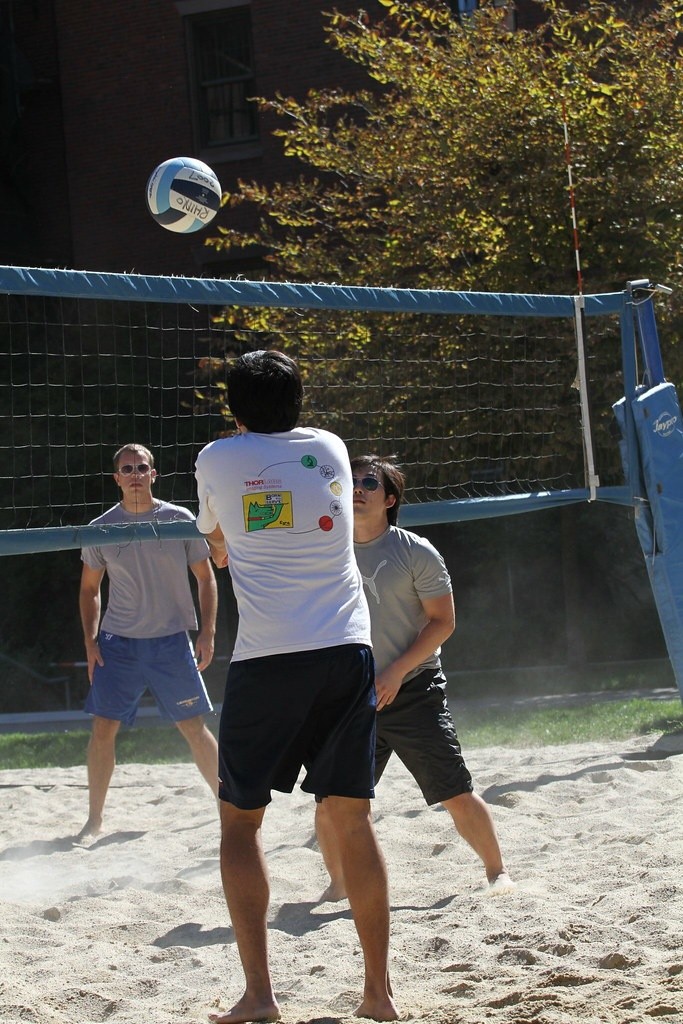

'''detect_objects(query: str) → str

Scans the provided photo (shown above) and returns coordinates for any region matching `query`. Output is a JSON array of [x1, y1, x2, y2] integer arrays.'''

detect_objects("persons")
[[315, 455, 502, 903], [77, 442, 220, 844], [194, 349, 399, 1024]]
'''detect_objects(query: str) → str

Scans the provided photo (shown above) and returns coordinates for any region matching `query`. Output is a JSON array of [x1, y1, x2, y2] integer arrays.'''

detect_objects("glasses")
[[118, 464, 150, 477], [353, 474, 386, 493]]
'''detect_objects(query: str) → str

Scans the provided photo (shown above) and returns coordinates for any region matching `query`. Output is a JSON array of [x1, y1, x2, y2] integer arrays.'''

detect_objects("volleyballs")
[[146, 155, 224, 234]]
